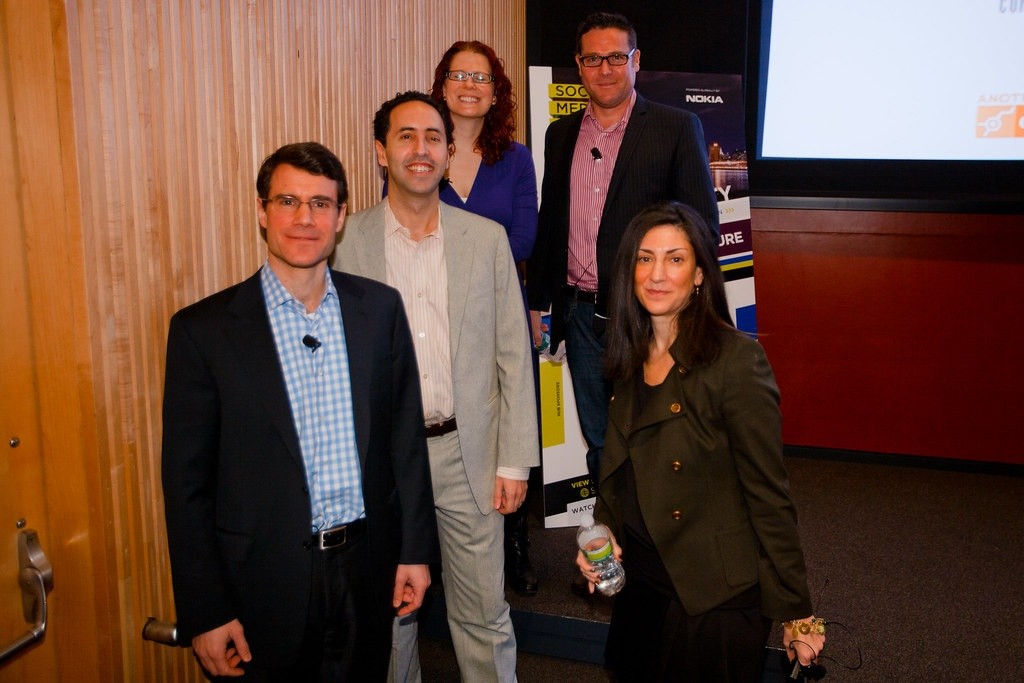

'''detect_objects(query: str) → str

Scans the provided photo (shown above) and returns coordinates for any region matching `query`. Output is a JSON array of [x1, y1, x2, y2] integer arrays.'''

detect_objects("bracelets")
[[782, 618, 826, 638]]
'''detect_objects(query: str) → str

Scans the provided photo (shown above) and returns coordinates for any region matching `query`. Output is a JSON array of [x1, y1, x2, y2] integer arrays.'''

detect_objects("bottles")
[[533, 329, 566, 363], [577, 514, 626, 596]]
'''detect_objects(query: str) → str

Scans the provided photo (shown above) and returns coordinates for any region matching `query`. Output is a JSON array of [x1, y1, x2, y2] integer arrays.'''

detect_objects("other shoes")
[[573, 574, 598, 595]]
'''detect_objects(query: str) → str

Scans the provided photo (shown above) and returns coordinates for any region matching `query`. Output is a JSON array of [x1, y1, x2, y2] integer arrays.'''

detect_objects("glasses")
[[263, 195, 339, 214], [578, 48, 634, 67], [445, 70, 494, 84]]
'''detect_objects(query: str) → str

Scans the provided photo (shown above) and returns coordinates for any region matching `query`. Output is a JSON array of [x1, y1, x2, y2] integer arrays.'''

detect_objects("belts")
[[423, 418, 456, 438], [313, 517, 366, 550]]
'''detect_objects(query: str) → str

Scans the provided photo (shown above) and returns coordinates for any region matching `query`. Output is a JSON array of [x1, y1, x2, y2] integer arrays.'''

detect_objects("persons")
[[383, 41, 539, 600], [162, 142, 440, 683], [331, 90, 542, 683], [576, 201, 827, 683], [526, 12, 721, 501]]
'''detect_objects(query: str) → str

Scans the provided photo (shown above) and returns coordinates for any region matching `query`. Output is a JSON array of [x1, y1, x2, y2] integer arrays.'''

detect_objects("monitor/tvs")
[[745, 0, 1024, 214]]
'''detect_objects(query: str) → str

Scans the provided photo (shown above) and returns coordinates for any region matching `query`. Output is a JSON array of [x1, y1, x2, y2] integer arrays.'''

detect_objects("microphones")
[[303, 335, 322, 354], [591, 147, 602, 160]]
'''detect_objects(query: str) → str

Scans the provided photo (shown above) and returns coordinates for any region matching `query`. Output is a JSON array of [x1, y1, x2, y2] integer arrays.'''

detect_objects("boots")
[[504, 498, 542, 595]]
